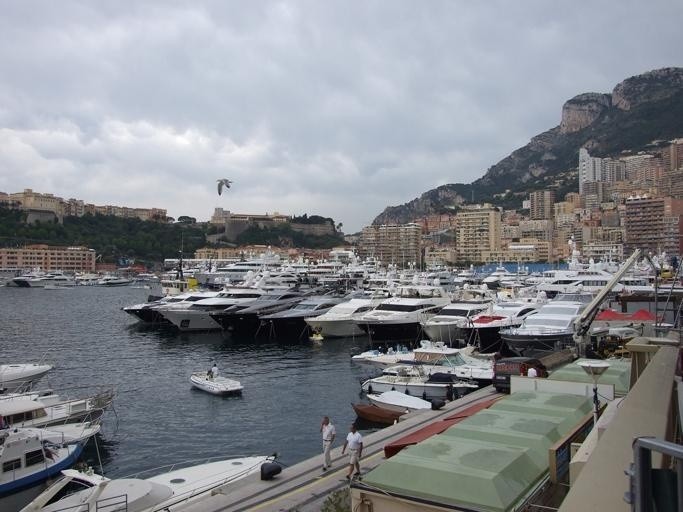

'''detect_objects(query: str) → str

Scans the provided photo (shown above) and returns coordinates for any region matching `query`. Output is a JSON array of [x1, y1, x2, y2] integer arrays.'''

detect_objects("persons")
[[341, 423, 363, 478], [210, 364, 218, 377], [604, 321, 648, 338], [519, 362, 547, 378], [587, 336, 620, 357], [377, 342, 407, 354], [319, 416, 335, 469]]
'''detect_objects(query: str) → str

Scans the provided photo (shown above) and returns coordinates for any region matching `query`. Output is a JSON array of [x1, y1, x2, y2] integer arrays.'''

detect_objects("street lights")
[[574, 355, 613, 426]]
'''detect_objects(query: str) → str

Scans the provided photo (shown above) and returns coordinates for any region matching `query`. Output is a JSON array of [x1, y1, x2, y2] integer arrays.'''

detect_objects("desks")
[[574, 355, 613, 426]]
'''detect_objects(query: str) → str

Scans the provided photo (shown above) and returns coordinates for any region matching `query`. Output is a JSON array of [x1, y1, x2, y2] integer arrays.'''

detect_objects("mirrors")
[[217, 179, 232, 195]]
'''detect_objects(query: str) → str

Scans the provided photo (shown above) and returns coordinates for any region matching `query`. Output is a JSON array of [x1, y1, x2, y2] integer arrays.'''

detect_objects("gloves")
[[11, 268, 157, 288], [366, 344, 414, 363], [0, 389, 112, 438], [365, 390, 434, 411], [350, 402, 402, 425], [0, 419, 102, 494], [351, 341, 537, 383], [20, 453, 277, 512], [0, 360, 52, 393], [190, 371, 243, 396], [360, 364, 481, 400]]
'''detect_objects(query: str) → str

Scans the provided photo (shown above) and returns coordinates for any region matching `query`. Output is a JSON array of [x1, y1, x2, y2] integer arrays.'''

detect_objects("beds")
[[626, 309, 661, 326], [592, 309, 623, 326]]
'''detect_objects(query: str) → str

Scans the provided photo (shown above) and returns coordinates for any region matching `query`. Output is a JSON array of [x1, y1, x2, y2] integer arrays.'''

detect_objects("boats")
[[365, 390, 434, 411], [0, 360, 52, 393], [0, 419, 102, 494], [11, 268, 157, 288], [0, 389, 112, 438], [360, 364, 481, 400], [120, 247, 683, 358], [366, 344, 414, 363], [190, 371, 243, 396], [350, 402, 402, 425], [351, 341, 537, 383], [20, 453, 277, 512]]
